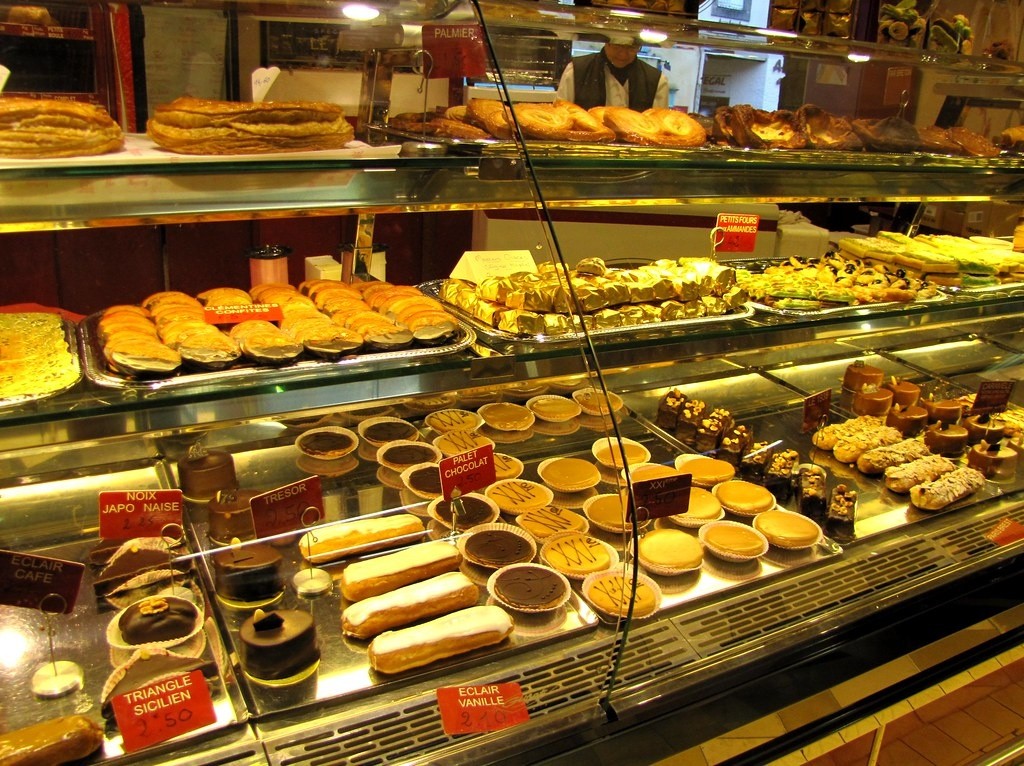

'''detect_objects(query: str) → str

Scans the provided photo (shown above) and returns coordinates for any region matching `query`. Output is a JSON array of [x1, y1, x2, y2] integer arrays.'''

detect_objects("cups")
[[250, 244, 290, 290]]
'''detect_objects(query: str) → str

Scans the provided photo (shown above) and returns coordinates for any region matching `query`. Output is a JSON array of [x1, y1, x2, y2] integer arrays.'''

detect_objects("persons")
[[554, 43, 668, 113]]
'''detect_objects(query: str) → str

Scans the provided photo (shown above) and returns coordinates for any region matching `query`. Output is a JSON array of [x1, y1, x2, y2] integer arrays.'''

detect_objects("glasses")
[[608, 42, 643, 53]]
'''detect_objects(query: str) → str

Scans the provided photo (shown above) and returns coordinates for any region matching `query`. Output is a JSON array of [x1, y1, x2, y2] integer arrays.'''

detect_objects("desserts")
[[98, 361, 1024, 680]]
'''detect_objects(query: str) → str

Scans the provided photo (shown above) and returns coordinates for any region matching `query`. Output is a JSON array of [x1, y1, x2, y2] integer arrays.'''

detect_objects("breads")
[[0, 96, 1024, 401]]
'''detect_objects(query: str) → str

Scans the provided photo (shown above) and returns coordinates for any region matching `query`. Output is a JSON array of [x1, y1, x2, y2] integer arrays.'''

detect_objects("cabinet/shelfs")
[[0, 0, 1024, 766]]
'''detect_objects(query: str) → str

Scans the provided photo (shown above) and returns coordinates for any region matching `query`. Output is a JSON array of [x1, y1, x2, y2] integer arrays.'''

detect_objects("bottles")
[[1013, 216, 1024, 254]]
